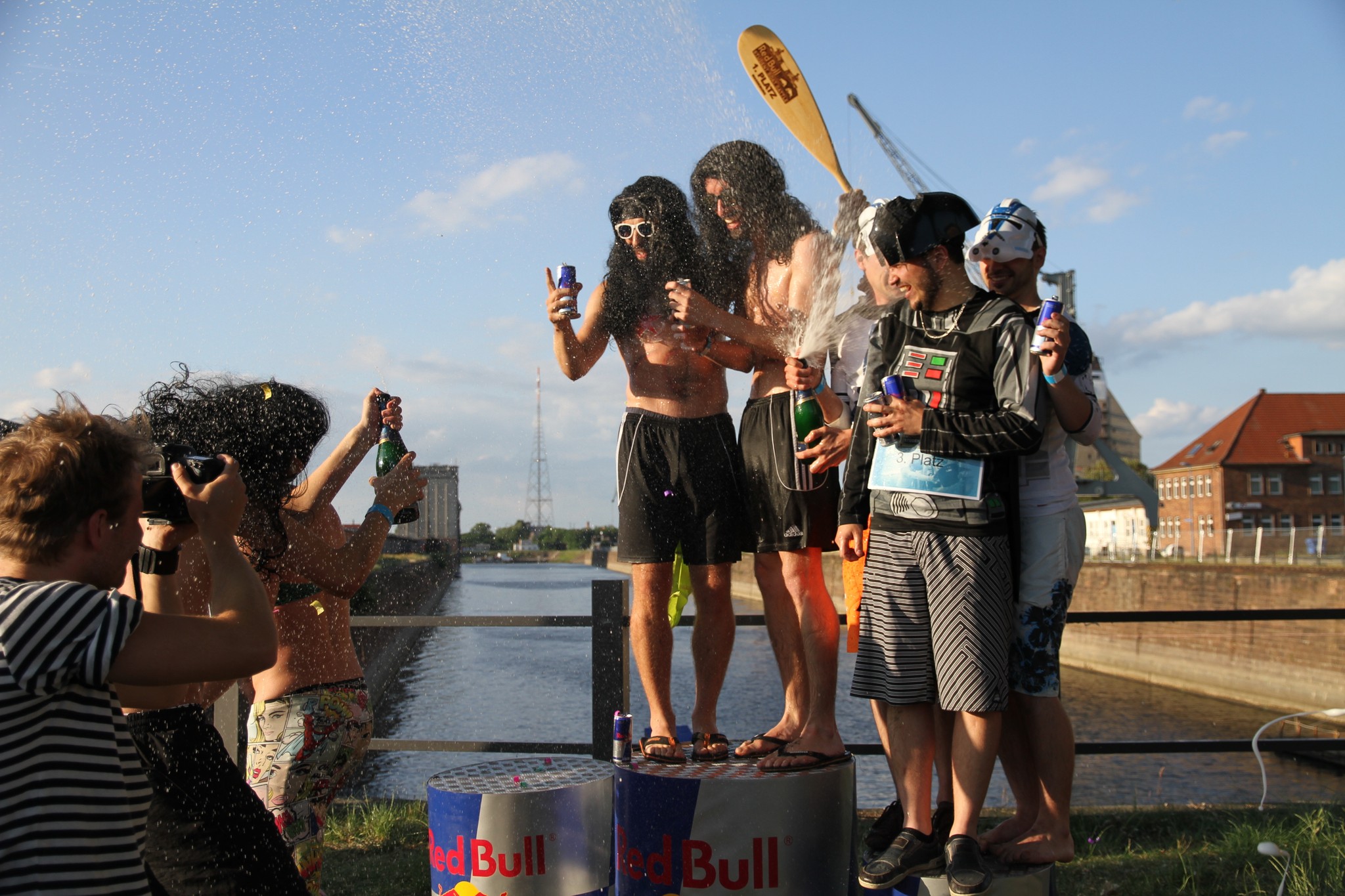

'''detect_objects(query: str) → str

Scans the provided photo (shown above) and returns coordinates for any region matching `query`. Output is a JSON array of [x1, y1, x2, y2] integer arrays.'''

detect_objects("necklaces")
[[919, 285, 976, 338]]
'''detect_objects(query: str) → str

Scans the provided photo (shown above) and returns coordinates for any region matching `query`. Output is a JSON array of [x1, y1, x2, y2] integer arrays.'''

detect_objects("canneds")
[[613, 711, 633, 764], [557, 262, 577, 314], [1030, 295, 1064, 356], [863, 391, 900, 447], [881, 373, 908, 401], [673, 278, 695, 328]]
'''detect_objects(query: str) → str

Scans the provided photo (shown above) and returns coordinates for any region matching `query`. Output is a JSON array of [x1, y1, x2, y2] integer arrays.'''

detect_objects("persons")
[[963, 197, 1102, 867], [0, 356, 429, 896], [546, 176, 739, 764], [664, 138, 852, 774], [785, 197, 954, 862], [832, 193, 1053, 896]]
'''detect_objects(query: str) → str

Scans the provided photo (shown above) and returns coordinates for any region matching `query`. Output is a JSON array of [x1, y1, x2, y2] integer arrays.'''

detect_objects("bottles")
[[375, 393, 421, 525], [793, 358, 825, 466]]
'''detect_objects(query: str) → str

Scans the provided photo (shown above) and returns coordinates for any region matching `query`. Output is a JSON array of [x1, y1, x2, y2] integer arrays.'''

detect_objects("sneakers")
[[944, 833, 993, 896], [931, 802, 979, 847], [866, 797, 904, 852], [858, 827, 944, 890]]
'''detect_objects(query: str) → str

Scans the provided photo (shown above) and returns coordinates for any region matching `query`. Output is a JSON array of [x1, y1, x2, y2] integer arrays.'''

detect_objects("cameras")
[[138, 441, 226, 523]]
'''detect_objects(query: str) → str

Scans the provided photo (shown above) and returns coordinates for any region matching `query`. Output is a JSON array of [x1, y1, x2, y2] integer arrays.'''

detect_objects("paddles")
[[738, 24, 853, 194]]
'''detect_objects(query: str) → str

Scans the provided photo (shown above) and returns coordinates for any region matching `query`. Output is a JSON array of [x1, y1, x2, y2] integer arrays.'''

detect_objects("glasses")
[[704, 189, 733, 209], [615, 222, 654, 239]]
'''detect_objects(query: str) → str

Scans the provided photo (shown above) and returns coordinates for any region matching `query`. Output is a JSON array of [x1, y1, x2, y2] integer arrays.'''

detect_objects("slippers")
[[639, 736, 687, 764], [691, 732, 729, 761], [734, 733, 791, 758], [757, 743, 851, 772]]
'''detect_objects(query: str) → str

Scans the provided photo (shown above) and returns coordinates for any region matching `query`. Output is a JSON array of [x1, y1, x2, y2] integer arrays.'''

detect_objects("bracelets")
[[1043, 363, 1068, 385], [811, 374, 827, 397], [695, 336, 713, 357], [135, 545, 180, 576], [366, 504, 394, 530]]
